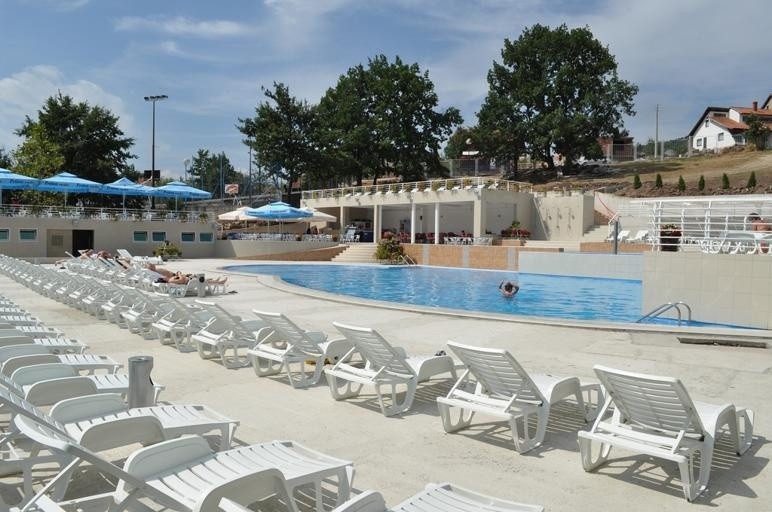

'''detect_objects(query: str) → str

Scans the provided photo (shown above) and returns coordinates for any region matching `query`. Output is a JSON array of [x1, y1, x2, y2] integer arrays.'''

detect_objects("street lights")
[[182, 158, 190, 181], [464, 137, 475, 176], [143, 94, 169, 209]]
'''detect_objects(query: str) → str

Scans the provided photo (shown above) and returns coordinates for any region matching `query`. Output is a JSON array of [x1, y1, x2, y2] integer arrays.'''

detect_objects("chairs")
[[4, 205, 218, 225], [576, 362, 755, 500], [328, 475, 550, 512], [230, 231, 334, 242], [435, 339, 588, 458], [608, 223, 755, 260], [1, 246, 356, 511], [324, 318, 481, 419]]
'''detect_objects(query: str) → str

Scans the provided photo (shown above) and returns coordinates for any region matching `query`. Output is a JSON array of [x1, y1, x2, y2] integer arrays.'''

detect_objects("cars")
[[514, 160, 608, 178]]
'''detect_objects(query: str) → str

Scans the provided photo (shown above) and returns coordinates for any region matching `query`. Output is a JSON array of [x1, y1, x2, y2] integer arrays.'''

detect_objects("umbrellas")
[[0, 167, 212, 215], [217, 201, 337, 235]]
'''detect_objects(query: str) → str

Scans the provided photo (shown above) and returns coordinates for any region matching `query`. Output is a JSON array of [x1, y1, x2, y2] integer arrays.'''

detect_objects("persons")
[[460, 230, 467, 237], [516, 229, 521, 235], [158, 200, 168, 223], [497, 280, 519, 302], [748, 212, 772, 253], [54, 248, 228, 284]]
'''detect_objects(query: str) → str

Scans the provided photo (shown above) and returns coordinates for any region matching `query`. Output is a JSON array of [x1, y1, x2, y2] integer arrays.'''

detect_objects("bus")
[[351, 177, 403, 192]]
